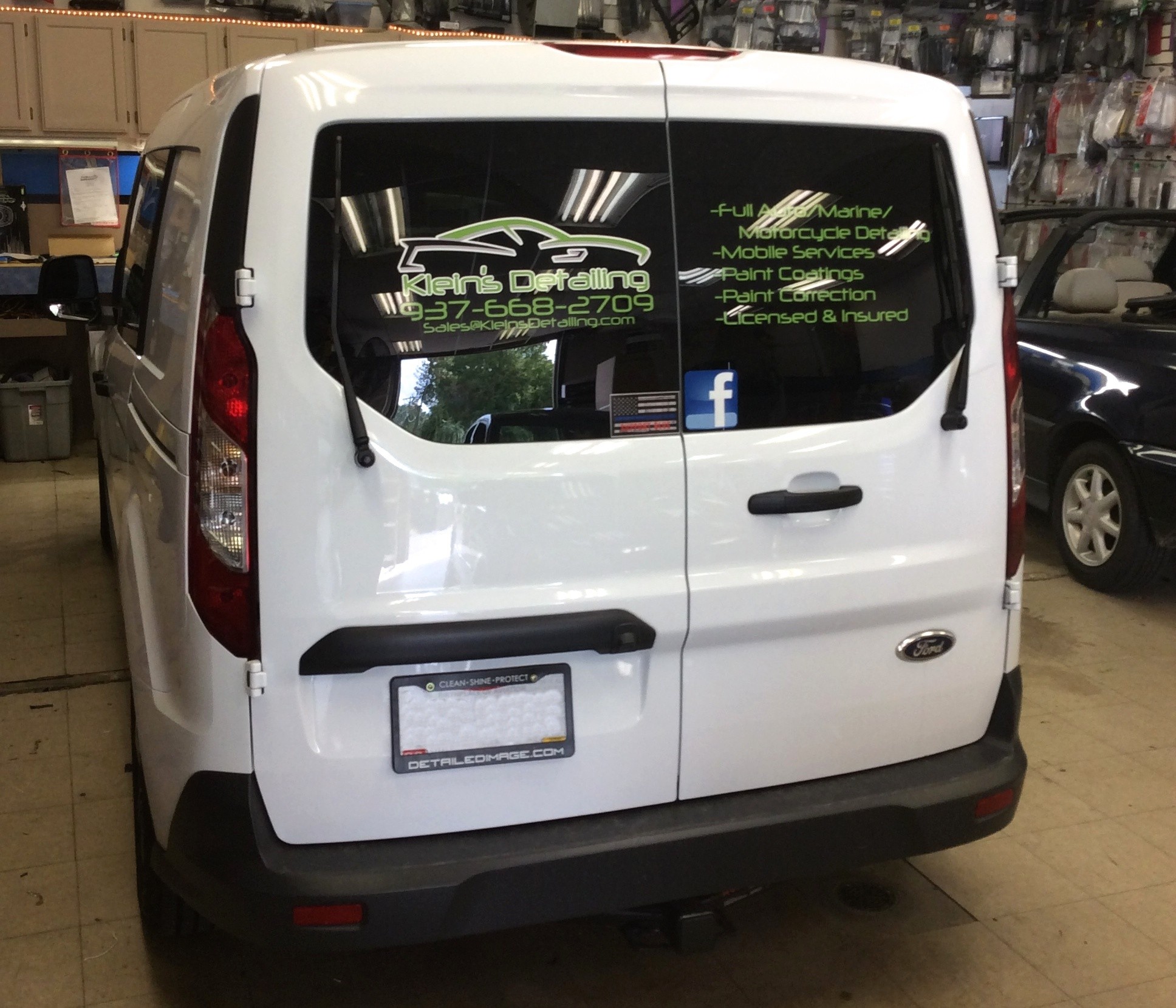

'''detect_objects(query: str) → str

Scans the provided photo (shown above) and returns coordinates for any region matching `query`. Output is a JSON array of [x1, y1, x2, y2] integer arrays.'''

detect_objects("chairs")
[[1038, 267, 1123, 326], [1093, 257, 1173, 312]]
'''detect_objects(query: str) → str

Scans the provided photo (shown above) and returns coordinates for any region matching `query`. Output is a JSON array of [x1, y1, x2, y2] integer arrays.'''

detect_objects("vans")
[[36, 33, 1038, 946]]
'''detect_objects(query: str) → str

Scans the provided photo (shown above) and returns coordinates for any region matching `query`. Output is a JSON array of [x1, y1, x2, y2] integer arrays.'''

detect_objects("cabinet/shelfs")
[[1, 6, 492, 139]]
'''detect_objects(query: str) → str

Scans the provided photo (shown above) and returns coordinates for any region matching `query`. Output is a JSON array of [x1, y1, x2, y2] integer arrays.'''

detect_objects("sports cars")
[[1000, 203, 1173, 588]]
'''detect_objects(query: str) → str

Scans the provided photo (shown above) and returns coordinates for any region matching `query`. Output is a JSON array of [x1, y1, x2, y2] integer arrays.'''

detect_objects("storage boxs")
[[1, 369, 74, 462]]
